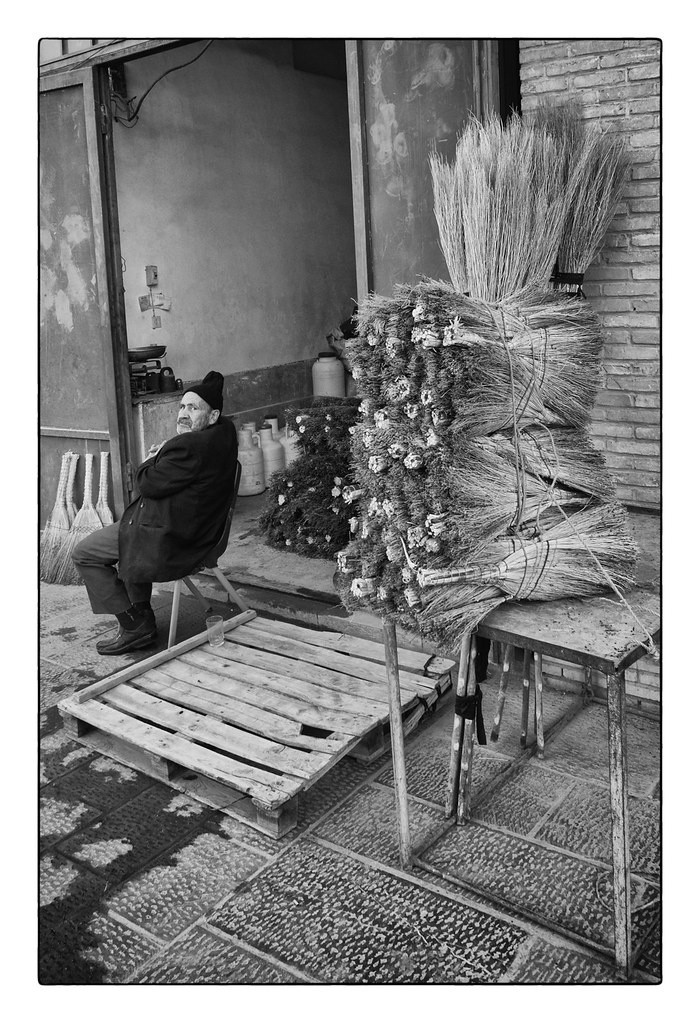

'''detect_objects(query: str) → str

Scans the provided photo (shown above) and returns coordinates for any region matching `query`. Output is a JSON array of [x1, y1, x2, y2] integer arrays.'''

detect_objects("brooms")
[[41, 451, 115, 587], [332, 94, 636, 826]]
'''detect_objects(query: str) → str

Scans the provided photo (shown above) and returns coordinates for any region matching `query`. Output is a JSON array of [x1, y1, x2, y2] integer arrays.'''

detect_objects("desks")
[[362, 507, 662, 983]]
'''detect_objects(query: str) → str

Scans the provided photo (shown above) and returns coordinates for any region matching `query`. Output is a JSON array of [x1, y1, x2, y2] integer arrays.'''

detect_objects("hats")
[[182, 370, 224, 413]]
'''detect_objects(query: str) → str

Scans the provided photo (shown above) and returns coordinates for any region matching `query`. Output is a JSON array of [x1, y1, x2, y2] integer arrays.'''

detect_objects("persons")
[[71, 371, 239, 657]]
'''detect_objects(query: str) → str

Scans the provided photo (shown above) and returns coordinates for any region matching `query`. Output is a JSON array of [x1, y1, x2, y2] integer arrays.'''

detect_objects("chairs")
[[166, 460, 249, 649]]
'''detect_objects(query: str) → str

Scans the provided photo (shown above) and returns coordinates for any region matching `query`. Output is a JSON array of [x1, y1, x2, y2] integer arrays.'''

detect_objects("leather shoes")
[[96, 619, 158, 654], [133, 603, 156, 624]]
